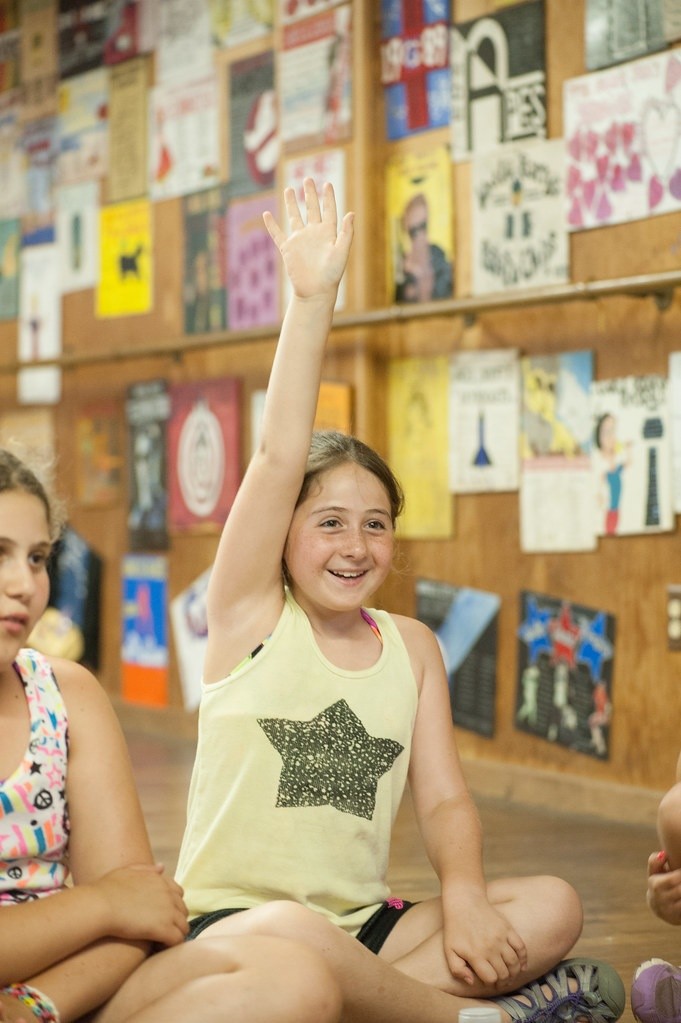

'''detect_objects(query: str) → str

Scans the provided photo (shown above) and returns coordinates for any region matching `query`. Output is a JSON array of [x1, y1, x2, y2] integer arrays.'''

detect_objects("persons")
[[176, 178, 625, 1023], [396, 196, 452, 301], [0, 452, 343, 1023], [632, 785, 681, 1023]]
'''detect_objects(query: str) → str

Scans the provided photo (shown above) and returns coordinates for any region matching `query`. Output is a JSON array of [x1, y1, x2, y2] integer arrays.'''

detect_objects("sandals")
[[489, 957, 625, 1023]]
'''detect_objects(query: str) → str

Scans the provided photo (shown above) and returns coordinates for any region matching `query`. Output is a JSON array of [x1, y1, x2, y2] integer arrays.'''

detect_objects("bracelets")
[[3, 983, 59, 1023]]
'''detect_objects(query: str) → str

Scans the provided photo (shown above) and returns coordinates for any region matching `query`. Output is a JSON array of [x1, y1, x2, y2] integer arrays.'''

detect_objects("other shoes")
[[630, 958, 681, 1023]]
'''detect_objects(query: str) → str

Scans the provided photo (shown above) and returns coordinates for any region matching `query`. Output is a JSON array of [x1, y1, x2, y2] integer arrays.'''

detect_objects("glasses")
[[410, 218, 427, 244]]
